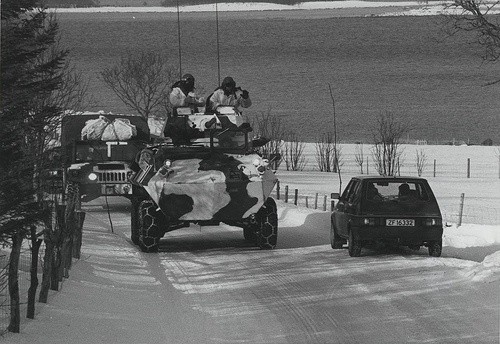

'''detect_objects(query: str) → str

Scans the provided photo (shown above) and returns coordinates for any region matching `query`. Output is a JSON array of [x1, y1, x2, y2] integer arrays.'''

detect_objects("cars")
[[329, 174, 444, 258]]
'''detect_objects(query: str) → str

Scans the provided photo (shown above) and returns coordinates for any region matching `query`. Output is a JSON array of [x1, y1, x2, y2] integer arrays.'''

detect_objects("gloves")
[[199, 97, 205, 103], [174, 89, 180, 95], [240, 90, 249, 99]]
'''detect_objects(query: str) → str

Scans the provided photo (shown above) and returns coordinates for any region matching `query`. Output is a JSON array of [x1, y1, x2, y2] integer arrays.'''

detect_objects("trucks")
[[37, 114, 151, 203]]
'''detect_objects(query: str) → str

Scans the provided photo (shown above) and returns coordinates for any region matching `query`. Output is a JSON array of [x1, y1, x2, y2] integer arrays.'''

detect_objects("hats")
[[180, 73, 195, 81], [222, 77, 236, 86]]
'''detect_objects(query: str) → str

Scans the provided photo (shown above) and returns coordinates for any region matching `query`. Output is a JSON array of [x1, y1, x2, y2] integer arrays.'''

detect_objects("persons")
[[399, 183, 410, 199], [170, 74, 206, 113], [209, 77, 258, 115]]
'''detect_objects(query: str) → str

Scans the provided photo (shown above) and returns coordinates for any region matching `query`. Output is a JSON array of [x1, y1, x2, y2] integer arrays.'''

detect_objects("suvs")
[[127, 102, 281, 251]]
